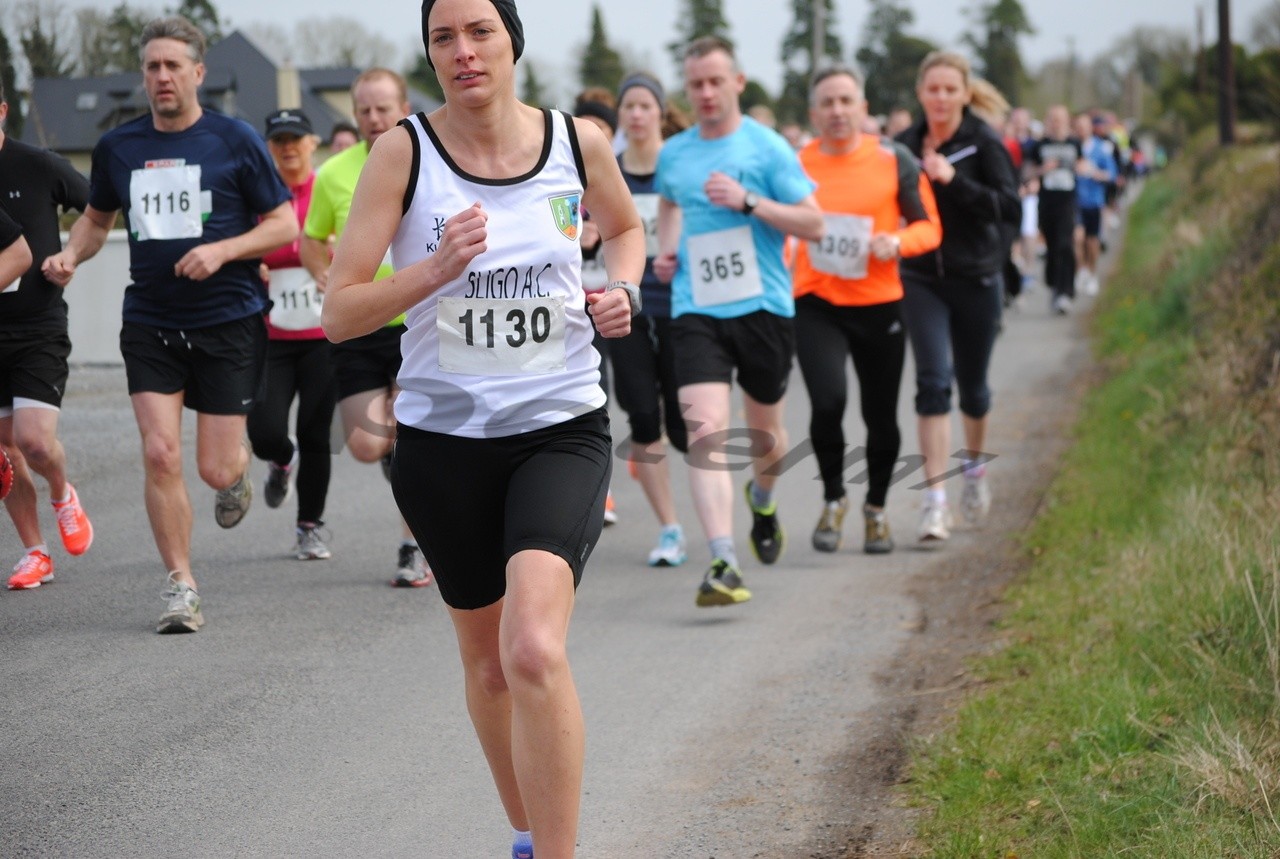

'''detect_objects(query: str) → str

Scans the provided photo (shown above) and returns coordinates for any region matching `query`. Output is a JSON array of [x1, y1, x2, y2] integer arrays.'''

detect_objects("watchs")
[[606, 281, 642, 318], [741, 192, 757, 215]]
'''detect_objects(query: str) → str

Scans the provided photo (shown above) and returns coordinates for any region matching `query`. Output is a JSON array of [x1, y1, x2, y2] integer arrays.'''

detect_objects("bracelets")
[[892, 235, 901, 256]]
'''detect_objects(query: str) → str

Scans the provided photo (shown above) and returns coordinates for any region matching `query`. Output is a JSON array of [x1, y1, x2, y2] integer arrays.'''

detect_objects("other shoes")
[[1087, 275, 1098, 295], [1055, 294, 1070, 314]]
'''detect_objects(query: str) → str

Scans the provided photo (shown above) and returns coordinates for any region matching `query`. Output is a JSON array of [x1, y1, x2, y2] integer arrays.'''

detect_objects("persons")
[[318, 0, 645, 859], [330, 124, 358, 157], [574, 33, 1147, 607], [41, 19, 300, 633], [1, 83, 92, 589], [245, 107, 335, 561], [300, 68, 435, 586]]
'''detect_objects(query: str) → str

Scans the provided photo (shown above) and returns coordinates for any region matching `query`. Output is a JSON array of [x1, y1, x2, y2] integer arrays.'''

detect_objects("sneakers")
[[917, 502, 955, 540], [381, 453, 392, 481], [649, 527, 686, 566], [812, 495, 851, 552], [214, 440, 253, 529], [155, 569, 205, 633], [601, 488, 617, 523], [863, 503, 892, 553], [695, 559, 751, 606], [745, 481, 782, 564], [391, 545, 430, 587], [263, 459, 290, 508], [52, 482, 93, 555], [291, 527, 335, 560], [627, 453, 639, 481], [960, 453, 990, 524], [8, 550, 54, 588]]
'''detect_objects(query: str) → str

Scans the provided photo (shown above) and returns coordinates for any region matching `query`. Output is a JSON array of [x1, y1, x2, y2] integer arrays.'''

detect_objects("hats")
[[263, 110, 311, 139]]
